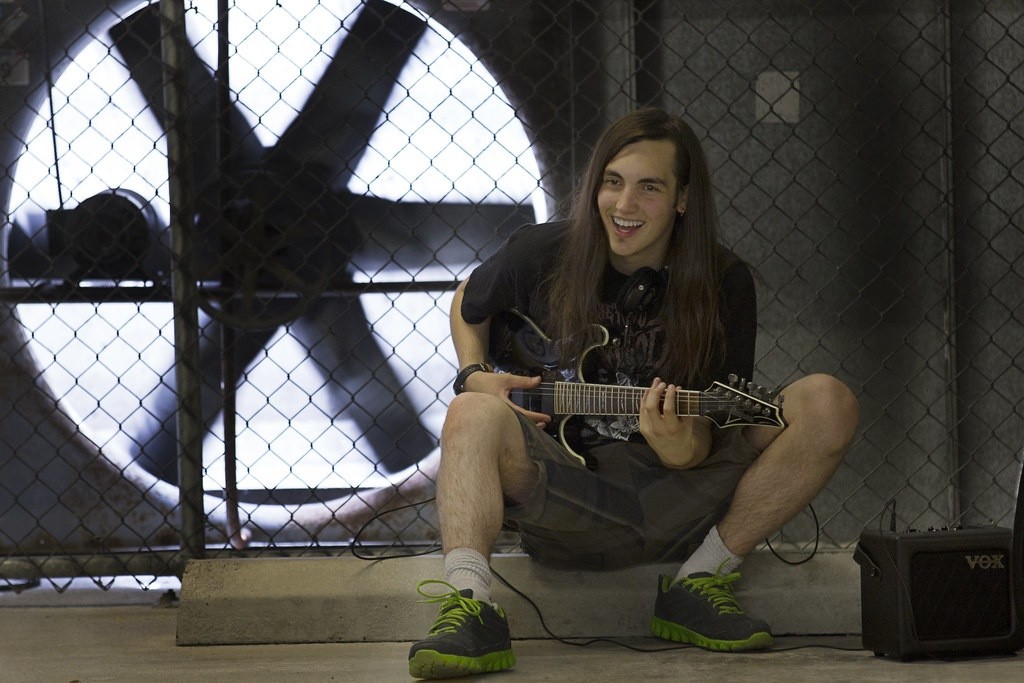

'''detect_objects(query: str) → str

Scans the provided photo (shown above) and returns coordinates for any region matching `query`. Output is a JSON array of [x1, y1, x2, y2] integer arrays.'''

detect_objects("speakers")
[[853, 524, 1024, 664]]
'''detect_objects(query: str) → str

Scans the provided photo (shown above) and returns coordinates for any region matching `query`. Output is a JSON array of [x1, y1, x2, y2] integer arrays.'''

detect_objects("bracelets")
[[453, 362, 489, 396]]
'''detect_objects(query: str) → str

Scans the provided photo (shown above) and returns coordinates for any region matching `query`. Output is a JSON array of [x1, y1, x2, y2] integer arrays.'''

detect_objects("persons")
[[406, 110, 863, 680]]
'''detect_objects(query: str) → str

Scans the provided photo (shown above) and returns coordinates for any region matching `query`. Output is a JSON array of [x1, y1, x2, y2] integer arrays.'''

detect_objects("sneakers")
[[650, 556, 775, 651], [408, 578, 515, 679]]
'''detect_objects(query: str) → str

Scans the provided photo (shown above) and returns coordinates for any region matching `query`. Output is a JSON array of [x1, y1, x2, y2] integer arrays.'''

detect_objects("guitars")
[[483, 305, 790, 473]]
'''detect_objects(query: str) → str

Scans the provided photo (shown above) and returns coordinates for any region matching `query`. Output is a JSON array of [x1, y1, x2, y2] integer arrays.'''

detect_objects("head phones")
[[615, 241, 676, 316]]
